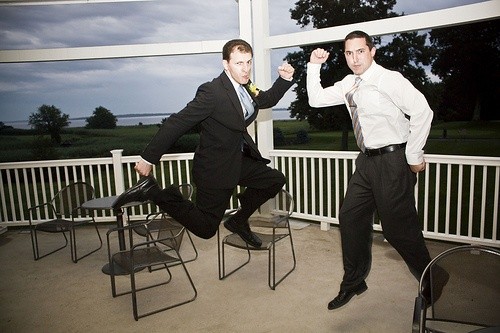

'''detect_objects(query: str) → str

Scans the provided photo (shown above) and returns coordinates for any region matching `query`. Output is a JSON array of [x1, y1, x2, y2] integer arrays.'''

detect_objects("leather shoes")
[[223, 216, 262, 248], [419, 265, 449, 310], [328, 280, 368, 310], [111, 172, 159, 209]]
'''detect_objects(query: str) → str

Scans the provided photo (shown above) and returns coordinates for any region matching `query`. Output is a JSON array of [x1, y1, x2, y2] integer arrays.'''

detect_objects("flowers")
[[248, 83, 259, 97]]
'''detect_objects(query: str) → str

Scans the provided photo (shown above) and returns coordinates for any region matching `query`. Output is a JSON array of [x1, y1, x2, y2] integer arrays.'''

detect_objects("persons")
[[110, 39, 296, 250], [306, 30, 449, 310]]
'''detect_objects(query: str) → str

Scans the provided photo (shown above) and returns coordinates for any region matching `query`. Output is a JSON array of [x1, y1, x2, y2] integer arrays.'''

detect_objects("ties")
[[238, 86, 255, 152], [344, 77, 367, 154]]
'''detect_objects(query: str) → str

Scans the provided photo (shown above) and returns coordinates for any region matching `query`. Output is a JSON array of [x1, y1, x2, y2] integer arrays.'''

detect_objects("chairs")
[[28, 182, 103, 264], [217, 188, 297, 290], [106, 212, 198, 321], [131, 183, 198, 271], [411, 245, 500, 333]]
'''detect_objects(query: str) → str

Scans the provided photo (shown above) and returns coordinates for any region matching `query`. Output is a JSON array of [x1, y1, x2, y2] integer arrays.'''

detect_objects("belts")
[[361, 142, 407, 157]]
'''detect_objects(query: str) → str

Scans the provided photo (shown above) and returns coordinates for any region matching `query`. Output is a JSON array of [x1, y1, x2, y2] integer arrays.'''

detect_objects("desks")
[[82, 196, 150, 275]]
